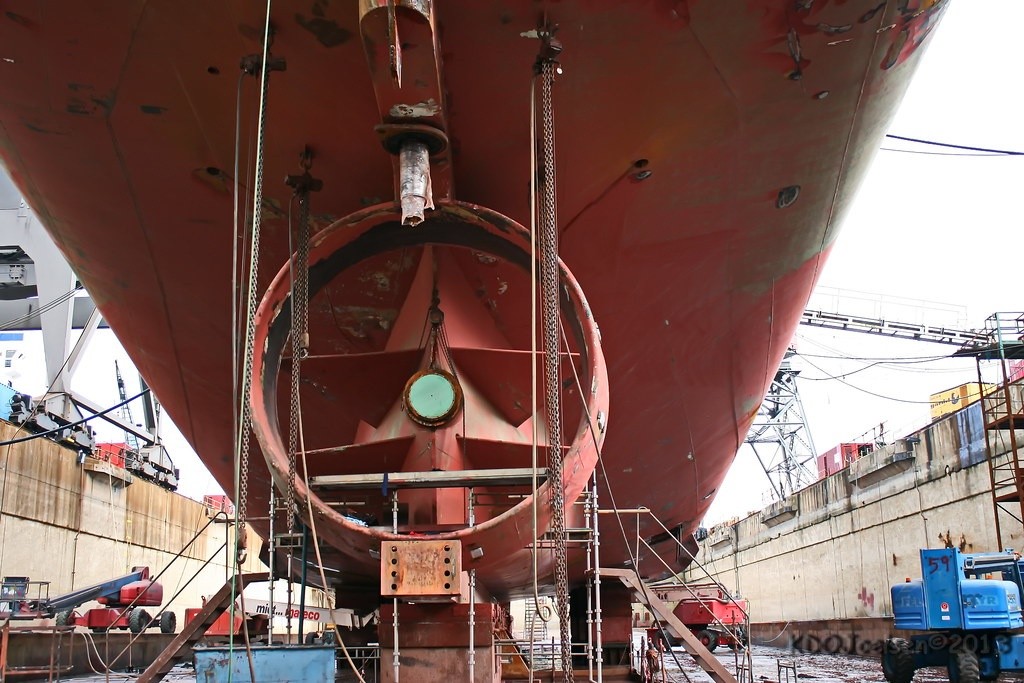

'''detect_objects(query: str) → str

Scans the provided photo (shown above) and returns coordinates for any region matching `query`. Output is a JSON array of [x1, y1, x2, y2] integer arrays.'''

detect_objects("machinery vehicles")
[[880, 546, 1024, 683], [0, 565, 176, 633], [647, 599, 746, 654]]
